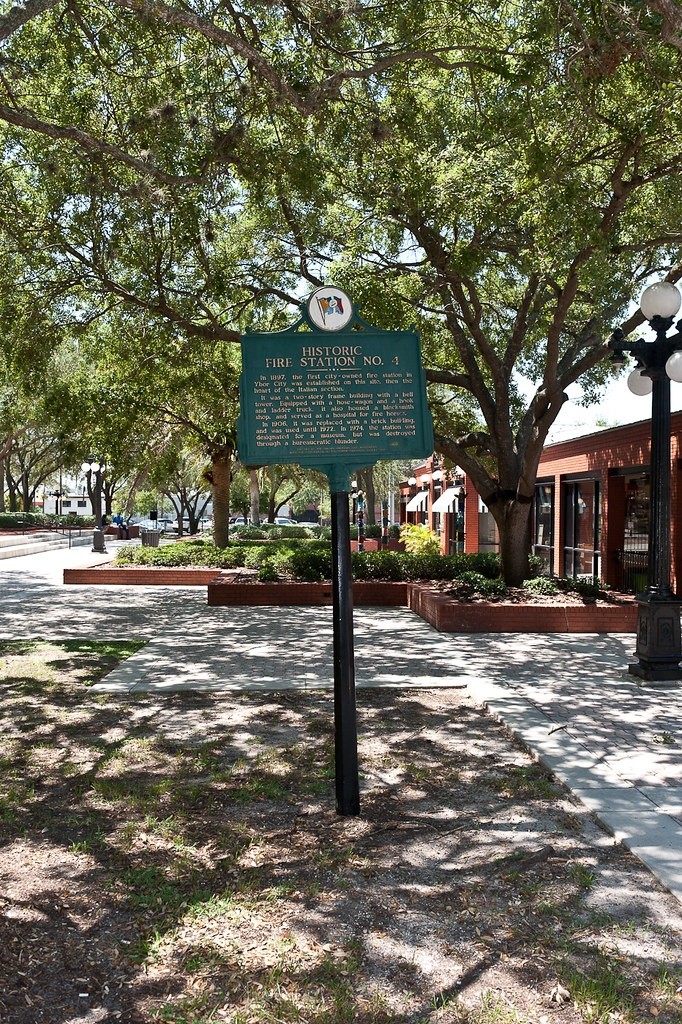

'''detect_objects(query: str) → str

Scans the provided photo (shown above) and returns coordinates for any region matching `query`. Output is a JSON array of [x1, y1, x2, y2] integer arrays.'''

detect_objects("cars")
[[129, 516, 322, 533]]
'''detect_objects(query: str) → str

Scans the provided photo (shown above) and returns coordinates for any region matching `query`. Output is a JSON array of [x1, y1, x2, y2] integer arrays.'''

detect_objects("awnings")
[[406, 490, 428, 512], [432, 484, 488, 513]]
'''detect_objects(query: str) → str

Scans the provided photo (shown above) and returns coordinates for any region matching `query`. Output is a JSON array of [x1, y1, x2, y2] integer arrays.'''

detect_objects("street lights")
[[81, 458, 105, 553], [608, 282, 682, 681], [351, 481, 363, 525]]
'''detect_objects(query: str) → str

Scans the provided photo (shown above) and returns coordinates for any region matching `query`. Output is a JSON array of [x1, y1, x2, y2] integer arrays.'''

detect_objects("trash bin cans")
[[141, 529, 160, 548]]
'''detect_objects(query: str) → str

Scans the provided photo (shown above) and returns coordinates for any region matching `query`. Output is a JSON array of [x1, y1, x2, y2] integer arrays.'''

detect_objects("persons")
[[113, 516, 131, 539], [289, 508, 293, 518], [101, 515, 109, 534], [307, 501, 314, 509]]
